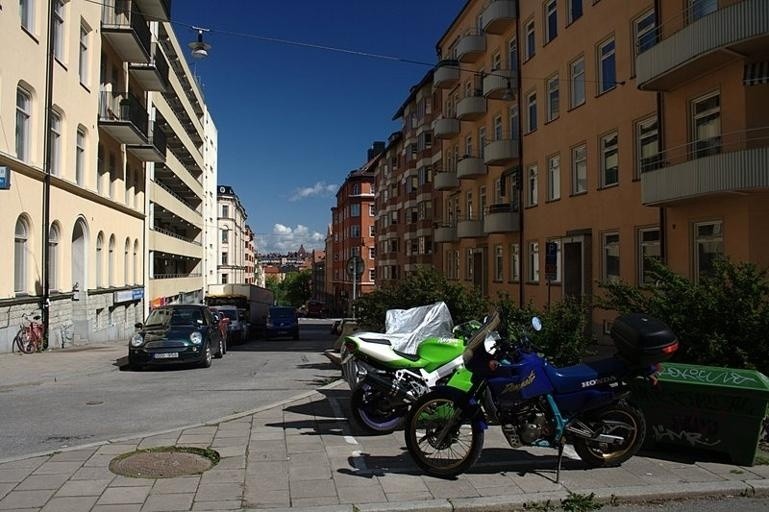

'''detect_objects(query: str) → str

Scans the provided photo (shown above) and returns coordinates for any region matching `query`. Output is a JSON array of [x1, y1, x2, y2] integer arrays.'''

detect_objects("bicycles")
[[16, 311, 44, 354]]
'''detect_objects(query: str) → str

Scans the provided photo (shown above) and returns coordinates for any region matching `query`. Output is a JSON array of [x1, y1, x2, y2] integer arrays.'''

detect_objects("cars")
[[128, 283, 329, 368]]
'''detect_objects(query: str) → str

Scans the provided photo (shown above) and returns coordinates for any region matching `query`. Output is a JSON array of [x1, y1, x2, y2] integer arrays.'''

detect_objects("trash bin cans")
[[648, 362, 769, 467]]
